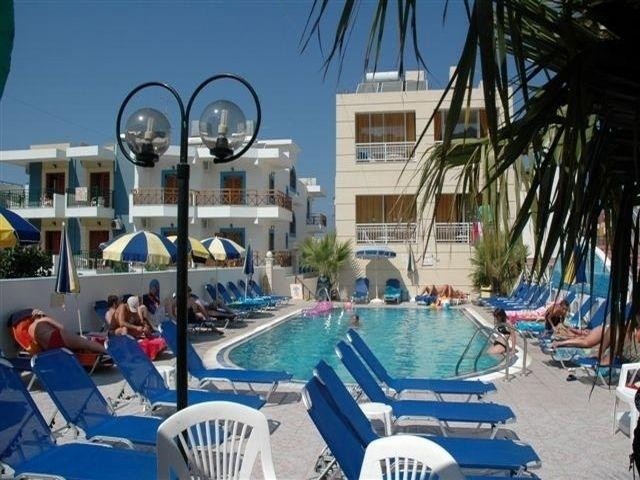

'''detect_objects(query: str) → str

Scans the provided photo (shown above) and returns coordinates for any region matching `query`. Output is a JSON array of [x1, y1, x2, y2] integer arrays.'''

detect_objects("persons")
[[348, 315, 359, 328], [419, 284, 468, 299], [488, 298, 639, 367], [29, 285, 236, 357]]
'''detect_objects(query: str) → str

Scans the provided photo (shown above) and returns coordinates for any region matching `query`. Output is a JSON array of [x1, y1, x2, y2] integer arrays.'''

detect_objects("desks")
[[358, 401, 398, 436]]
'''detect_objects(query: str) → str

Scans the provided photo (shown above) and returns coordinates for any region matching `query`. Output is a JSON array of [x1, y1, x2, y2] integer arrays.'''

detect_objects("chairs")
[[0, 275, 283, 325], [0, 355, 178, 478], [30, 348, 231, 457], [300, 377, 539, 478], [312, 359, 544, 478], [472, 273, 640, 439], [334, 340, 517, 439], [359, 432, 466, 478], [343, 328, 500, 401], [155, 399, 276, 479], [157, 321, 293, 406], [104, 333, 268, 417], [313, 273, 464, 305]]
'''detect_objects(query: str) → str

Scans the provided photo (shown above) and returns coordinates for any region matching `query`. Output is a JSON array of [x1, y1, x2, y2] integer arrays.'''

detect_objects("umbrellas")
[[241, 239, 253, 300], [356, 246, 397, 298], [199, 235, 246, 310], [166, 235, 210, 264], [55, 221, 83, 337], [103, 230, 178, 304], [0, 208, 41, 247]]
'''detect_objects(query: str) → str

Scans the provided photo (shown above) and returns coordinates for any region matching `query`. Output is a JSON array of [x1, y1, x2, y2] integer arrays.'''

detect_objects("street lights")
[[113, 72, 262, 469]]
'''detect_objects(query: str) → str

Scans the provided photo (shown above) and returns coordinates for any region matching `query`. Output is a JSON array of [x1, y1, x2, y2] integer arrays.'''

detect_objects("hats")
[[126, 295, 140, 314]]
